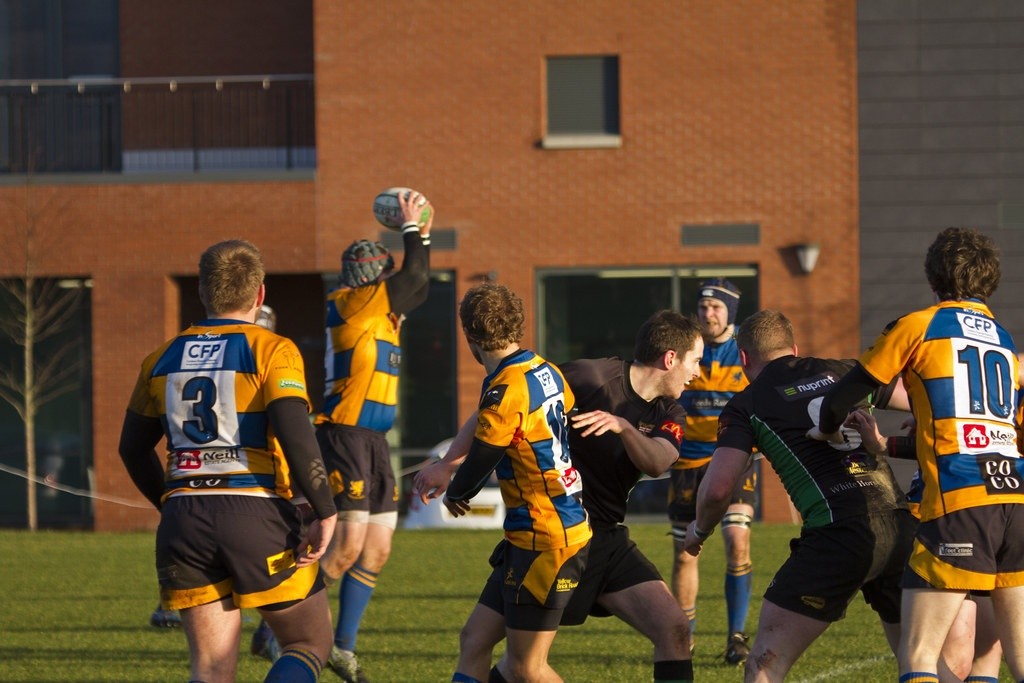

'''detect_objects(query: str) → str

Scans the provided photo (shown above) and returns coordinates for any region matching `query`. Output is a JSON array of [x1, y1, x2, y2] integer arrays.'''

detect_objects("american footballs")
[[372, 187, 431, 233]]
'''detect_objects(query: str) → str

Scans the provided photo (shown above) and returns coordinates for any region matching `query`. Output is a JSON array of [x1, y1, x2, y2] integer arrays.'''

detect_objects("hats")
[[697, 279, 741, 325]]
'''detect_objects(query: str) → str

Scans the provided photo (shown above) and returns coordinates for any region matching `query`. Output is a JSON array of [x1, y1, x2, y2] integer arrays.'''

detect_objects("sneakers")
[[150, 609, 182, 628], [689, 643, 696, 656], [328, 646, 367, 683], [252, 624, 280, 663], [725, 635, 751, 666]]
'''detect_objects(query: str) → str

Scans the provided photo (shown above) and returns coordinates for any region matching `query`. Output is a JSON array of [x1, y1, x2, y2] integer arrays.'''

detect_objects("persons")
[[667, 279, 766, 664], [150, 301, 282, 662], [683, 226, 1024, 683], [414, 284, 705, 683], [252, 188, 434, 683], [119, 238, 338, 683]]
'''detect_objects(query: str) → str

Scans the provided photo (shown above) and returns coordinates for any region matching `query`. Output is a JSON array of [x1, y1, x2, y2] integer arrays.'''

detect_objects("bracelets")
[[694, 526, 709, 539]]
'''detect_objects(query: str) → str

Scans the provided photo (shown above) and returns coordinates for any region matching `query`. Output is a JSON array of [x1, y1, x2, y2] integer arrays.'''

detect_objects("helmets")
[[342, 239, 393, 288]]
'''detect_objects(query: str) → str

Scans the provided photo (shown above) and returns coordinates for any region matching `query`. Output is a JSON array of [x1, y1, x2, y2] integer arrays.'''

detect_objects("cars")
[[404, 439, 507, 530]]
